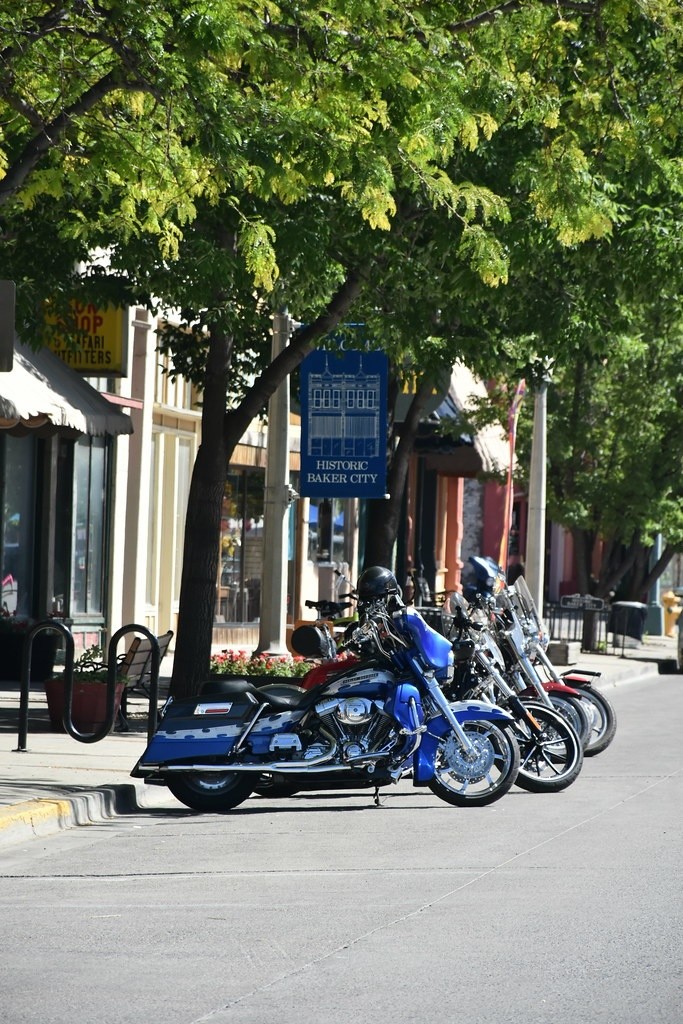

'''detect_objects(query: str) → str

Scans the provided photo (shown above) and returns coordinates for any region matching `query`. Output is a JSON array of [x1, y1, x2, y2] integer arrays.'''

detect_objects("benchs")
[[80, 631, 175, 732]]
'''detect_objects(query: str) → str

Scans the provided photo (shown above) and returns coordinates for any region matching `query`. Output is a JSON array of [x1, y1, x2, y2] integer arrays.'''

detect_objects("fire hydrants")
[[662, 592, 682, 638]]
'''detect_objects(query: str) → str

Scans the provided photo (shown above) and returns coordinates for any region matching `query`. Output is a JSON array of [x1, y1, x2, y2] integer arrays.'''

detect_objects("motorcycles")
[[130, 555, 615, 813]]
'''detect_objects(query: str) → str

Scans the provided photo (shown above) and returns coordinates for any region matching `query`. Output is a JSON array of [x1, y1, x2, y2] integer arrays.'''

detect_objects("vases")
[[211, 674, 303, 688]]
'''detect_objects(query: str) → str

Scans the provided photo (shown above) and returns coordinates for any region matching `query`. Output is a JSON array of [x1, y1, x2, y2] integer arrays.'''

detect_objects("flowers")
[[221, 481, 252, 562], [209, 649, 352, 677]]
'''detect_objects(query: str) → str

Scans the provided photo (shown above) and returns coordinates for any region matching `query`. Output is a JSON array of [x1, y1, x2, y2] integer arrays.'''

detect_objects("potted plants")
[[45, 643, 130, 733]]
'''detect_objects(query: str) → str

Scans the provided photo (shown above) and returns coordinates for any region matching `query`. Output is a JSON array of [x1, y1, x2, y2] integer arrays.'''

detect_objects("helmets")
[[357, 566, 397, 597]]
